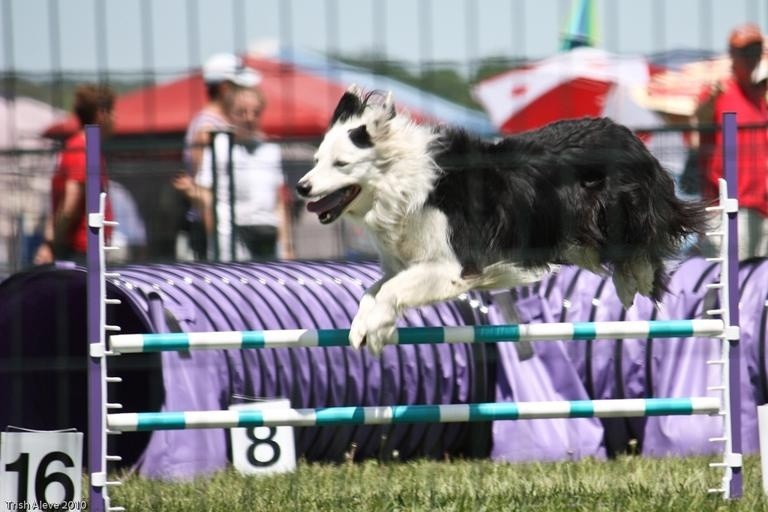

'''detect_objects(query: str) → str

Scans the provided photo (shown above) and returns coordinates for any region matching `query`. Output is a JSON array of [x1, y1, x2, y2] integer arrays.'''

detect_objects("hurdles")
[[86, 112, 744, 512]]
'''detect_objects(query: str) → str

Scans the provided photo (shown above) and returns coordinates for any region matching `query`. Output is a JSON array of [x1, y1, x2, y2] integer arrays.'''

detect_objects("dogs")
[[295, 83, 722, 360]]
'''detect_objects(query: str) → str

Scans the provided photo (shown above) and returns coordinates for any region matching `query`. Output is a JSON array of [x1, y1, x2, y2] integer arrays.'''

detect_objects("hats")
[[202, 52, 263, 88], [730, 25, 764, 50]]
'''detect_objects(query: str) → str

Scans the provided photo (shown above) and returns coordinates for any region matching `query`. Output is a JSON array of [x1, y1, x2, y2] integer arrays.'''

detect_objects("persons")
[[172, 49, 262, 265], [174, 90, 298, 260], [681, 26, 768, 258], [18, 121, 374, 264], [29, 84, 114, 268]]
